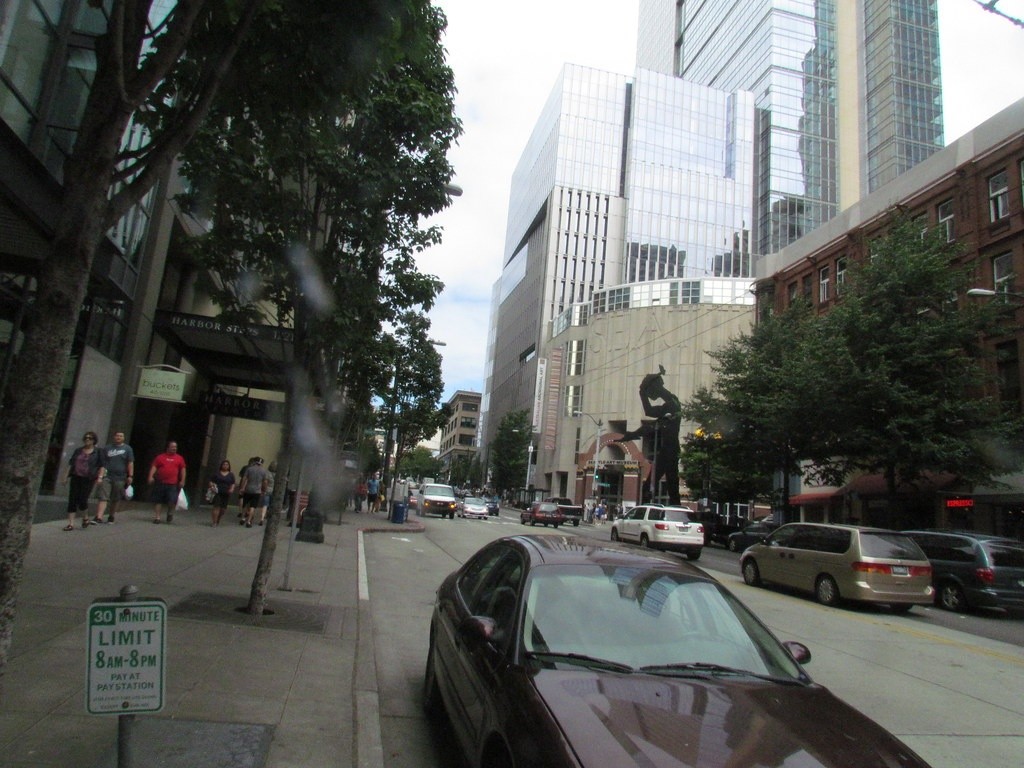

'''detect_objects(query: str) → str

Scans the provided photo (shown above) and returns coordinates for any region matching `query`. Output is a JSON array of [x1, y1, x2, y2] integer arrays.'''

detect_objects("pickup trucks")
[[544, 497, 584, 526]]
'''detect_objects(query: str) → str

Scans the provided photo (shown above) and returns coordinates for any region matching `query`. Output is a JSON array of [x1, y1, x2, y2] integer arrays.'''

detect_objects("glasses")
[[84, 437, 94, 440]]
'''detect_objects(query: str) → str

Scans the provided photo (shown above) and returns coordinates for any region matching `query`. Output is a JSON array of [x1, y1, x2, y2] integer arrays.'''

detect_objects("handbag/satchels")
[[124, 484, 134, 501], [174, 487, 188, 511]]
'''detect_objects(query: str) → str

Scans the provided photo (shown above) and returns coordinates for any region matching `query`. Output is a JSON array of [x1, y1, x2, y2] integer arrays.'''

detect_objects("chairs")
[[659, 582, 770, 675]]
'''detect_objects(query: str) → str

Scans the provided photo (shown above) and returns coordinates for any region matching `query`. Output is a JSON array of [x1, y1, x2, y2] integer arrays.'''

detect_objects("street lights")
[[379, 337, 447, 512], [649, 413, 672, 504], [512, 430, 534, 505], [573, 410, 603, 500]]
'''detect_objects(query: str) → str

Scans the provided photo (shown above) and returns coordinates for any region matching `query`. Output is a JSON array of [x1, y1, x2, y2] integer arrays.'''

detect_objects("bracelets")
[[128, 475, 133, 477], [98, 476, 103, 478]]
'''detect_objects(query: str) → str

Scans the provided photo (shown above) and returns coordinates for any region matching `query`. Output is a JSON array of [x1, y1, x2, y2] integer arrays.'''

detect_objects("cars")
[[452, 486, 500, 520], [423, 531, 932, 768], [398, 476, 422, 508], [520, 501, 566, 528], [693, 511, 784, 553]]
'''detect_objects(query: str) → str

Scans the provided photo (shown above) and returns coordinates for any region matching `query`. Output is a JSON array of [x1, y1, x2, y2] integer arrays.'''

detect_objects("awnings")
[[790, 493, 837, 504], [834, 470, 960, 498]]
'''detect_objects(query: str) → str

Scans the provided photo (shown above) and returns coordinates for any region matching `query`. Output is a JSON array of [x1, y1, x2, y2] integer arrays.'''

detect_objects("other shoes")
[[259, 520, 263, 526], [64, 525, 73, 531], [240, 520, 245, 525], [237, 513, 242, 518], [167, 515, 173, 523], [210, 521, 218, 527], [246, 521, 252, 528], [107, 516, 115, 525], [90, 517, 102, 524], [82, 519, 89, 528], [154, 518, 160, 524]]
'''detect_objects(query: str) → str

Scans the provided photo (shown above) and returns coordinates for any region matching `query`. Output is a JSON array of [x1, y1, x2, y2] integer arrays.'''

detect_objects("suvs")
[[902, 528, 1024, 614], [611, 503, 704, 560], [740, 521, 936, 615], [416, 484, 458, 519]]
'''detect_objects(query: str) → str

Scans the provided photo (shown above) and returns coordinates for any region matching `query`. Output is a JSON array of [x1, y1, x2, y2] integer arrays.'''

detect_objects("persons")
[[148, 440, 186, 524], [63, 432, 105, 531], [352, 472, 367, 513], [367, 471, 384, 514], [91, 432, 134, 524], [591, 503, 607, 524], [237, 457, 277, 526], [208, 460, 236, 527]]
